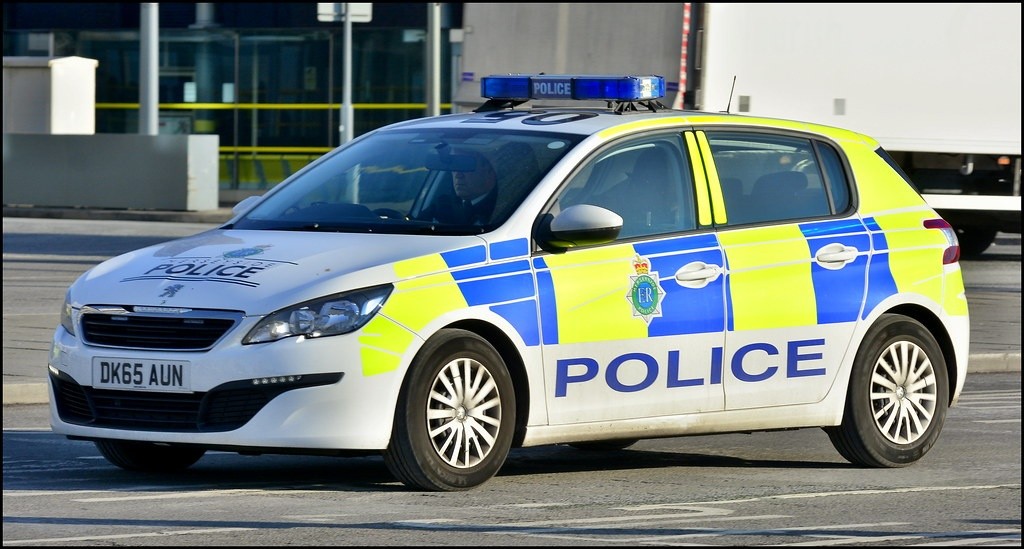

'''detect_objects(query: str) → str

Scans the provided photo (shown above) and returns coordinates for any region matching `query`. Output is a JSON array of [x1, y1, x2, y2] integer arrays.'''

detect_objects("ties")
[[463, 201, 472, 211]]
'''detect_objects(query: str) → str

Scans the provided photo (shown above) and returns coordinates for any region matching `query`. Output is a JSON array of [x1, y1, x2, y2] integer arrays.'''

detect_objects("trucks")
[[450, 2, 1021, 262]]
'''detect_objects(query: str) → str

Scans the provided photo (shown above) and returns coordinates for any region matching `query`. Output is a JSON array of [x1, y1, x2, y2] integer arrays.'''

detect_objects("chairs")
[[488, 143, 540, 224], [590, 147, 675, 238], [719, 171, 808, 224]]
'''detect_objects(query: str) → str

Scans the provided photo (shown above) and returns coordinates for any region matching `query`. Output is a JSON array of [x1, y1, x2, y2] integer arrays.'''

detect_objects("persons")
[[406, 148, 500, 228]]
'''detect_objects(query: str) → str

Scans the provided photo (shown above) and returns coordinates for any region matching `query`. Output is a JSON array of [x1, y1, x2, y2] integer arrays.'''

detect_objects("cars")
[[45, 68, 972, 494]]
